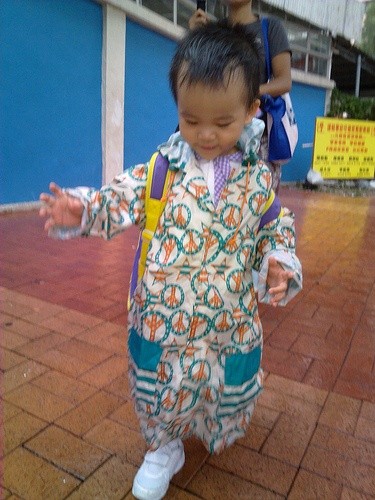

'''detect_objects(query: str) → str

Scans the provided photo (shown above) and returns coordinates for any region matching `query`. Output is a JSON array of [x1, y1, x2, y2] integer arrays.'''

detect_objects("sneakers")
[[132, 439, 185, 500]]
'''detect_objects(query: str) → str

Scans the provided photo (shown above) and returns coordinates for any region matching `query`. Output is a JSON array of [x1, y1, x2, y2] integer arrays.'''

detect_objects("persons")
[[187, 1, 292, 195], [38, 18, 303, 500]]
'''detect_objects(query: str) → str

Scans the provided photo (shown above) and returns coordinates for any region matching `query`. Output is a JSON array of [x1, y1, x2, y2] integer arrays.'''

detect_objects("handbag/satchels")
[[262, 92, 298, 165]]
